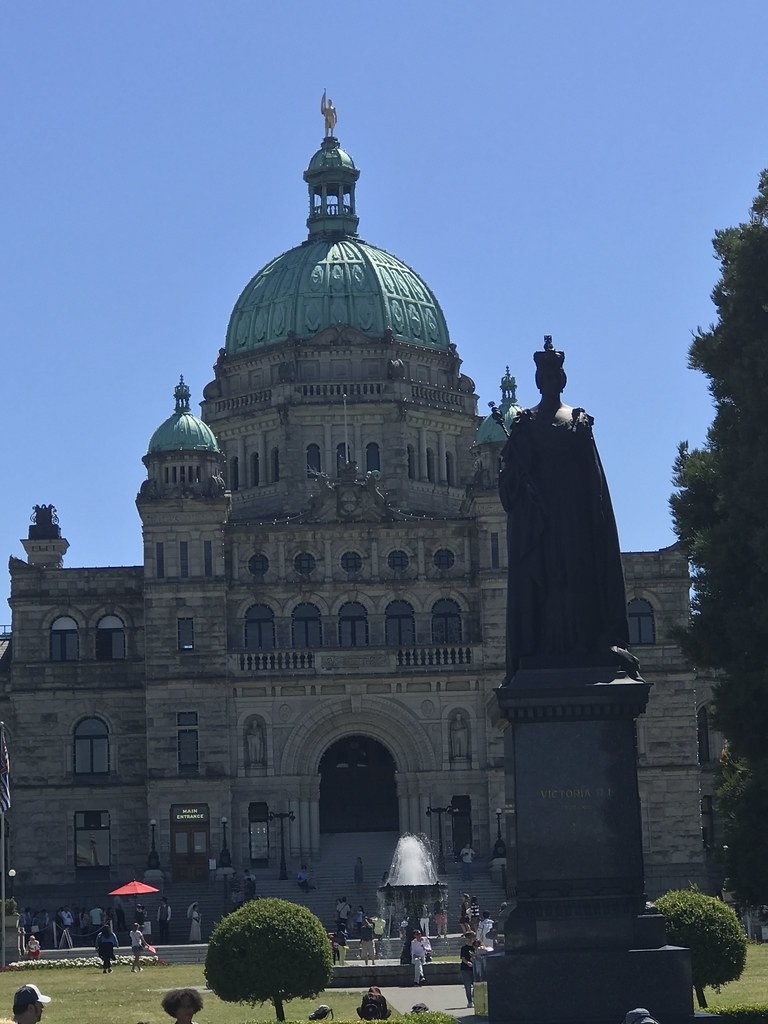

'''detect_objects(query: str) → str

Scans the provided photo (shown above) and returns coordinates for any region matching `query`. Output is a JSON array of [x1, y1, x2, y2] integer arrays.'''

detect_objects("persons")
[[497, 363, 639, 676], [354, 857, 364, 893], [246, 719, 264, 762], [161, 988, 204, 1024], [460, 843, 476, 882], [451, 713, 468, 759], [410, 929, 432, 987], [434, 895, 448, 940], [157, 897, 171, 944], [11, 984, 44, 1024], [459, 893, 507, 1007], [297, 864, 320, 895], [321, 93, 337, 137], [231, 869, 256, 909], [96, 925, 119, 974], [19, 904, 127, 947], [416, 903, 432, 937], [28, 935, 41, 960], [752, 909, 763, 945], [382, 872, 389, 882], [129, 903, 149, 973], [328, 896, 353, 967], [189, 905, 202, 943], [357, 906, 386, 965]]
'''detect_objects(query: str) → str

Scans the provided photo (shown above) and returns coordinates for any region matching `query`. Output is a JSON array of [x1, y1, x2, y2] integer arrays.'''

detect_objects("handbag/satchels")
[[140, 945, 145, 954]]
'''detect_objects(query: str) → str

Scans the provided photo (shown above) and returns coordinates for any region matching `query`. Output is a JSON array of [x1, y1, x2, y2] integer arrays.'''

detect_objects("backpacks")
[[309, 1004, 334, 1021]]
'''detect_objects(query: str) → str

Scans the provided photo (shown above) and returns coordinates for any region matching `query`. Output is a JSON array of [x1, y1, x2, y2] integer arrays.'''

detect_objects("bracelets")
[[145, 943, 148, 945]]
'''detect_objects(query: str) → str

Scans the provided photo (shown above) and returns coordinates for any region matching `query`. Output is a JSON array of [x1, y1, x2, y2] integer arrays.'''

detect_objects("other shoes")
[[108, 970, 111, 972], [414, 982, 420, 986], [103, 970, 107, 973], [421, 980, 427, 983], [131, 969, 137, 972], [467, 1002, 474, 1008], [139, 968, 143, 971]]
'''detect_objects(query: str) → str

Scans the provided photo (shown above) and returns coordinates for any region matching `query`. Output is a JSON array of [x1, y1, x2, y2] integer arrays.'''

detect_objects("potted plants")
[[0, 898, 20, 928]]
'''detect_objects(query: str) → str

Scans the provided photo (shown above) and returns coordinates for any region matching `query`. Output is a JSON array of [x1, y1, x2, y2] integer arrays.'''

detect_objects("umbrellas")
[[186, 901, 200, 919], [107, 876, 160, 910]]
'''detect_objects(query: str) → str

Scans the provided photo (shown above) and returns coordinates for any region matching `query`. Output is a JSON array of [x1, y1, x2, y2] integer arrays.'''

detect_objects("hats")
[[14, 983, 51, 1006]]
